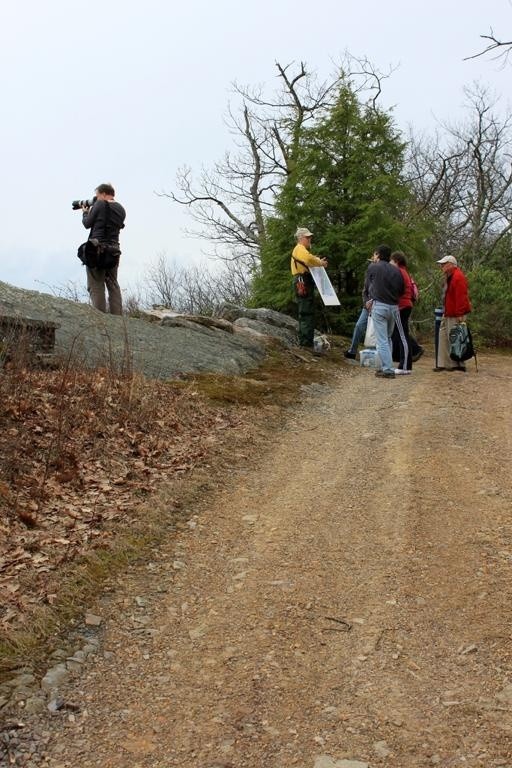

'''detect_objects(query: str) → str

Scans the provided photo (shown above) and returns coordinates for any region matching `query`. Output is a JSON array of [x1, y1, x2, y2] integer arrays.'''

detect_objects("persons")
[[432, 255, 473, 373], [344, 244, 425, 379], [72, 184, 126, 317], [290, 227, 329, 356]]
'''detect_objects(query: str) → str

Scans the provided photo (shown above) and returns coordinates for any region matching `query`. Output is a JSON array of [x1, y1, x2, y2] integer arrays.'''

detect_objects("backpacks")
[[410, 273, 418, 307], [446, 321, 475, 363]]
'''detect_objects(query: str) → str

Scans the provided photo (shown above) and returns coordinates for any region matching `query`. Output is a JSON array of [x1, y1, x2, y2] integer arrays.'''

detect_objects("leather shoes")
[[344, 350, 356, 359]]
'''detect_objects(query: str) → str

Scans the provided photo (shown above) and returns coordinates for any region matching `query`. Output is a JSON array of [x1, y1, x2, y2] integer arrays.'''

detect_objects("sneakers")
[[374, 366, 412, 379], [411, 345, 425, 362]]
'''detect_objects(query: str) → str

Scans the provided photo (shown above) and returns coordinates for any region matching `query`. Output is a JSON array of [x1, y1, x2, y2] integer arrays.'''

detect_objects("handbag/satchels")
[[77, 238, 121, 270], [296, 280, 314, 298]]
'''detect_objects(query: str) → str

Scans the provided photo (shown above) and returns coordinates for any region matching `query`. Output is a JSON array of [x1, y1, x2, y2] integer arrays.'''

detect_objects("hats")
[[436, 255, 457, 266], [294, 227, 314, 237]]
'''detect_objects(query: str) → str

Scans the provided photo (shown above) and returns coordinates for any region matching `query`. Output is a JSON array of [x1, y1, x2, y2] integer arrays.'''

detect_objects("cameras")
[[71, 196, 97, 210]]
